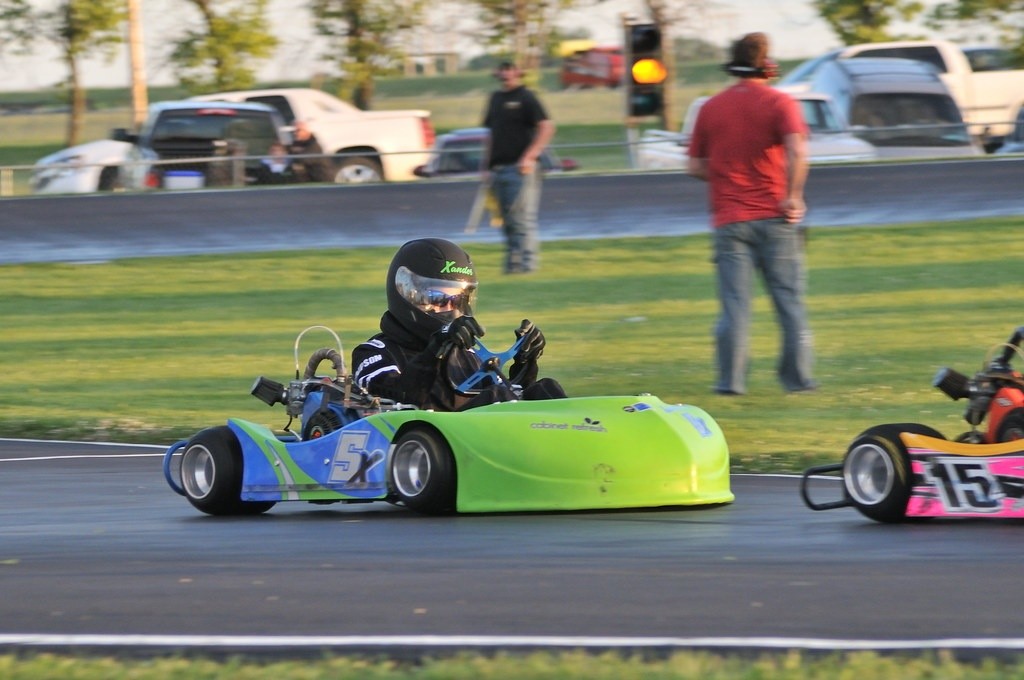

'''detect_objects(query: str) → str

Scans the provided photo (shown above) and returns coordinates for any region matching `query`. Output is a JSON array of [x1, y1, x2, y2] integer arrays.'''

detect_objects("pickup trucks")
[[111, 101, 305, 190], [777, 39, 1024, 152], [637, 89, 881, 168]]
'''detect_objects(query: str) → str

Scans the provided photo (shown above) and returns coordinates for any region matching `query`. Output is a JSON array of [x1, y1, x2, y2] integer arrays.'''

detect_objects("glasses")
[[419, 288, 470, 311]]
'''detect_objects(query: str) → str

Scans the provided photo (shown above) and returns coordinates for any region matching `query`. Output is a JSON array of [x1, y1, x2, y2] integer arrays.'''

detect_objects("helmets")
[[386, 239, 475, 342]]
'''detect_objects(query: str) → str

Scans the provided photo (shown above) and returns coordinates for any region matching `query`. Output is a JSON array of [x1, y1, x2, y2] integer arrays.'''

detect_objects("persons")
[[350, 237, 568, 412], [288, 121, 338, 184], [248, 144, 300, 185], [687, 32, 824, 394], [479, 61, 556, 276]]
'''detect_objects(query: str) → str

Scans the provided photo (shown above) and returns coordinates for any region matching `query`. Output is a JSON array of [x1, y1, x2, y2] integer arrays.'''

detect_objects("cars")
[[809, 57, 976, 158], [29, 86, 438, 197], [413, 126, 579, 178]]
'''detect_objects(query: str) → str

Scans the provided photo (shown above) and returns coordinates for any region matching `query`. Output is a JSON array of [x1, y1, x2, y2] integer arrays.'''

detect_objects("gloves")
[[515, 319, 544, 365], [445, 315, 482, 350]]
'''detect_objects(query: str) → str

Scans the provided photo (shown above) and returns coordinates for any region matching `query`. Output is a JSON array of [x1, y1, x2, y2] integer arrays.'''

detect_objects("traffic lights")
[[624, 22, 667, 116]]
